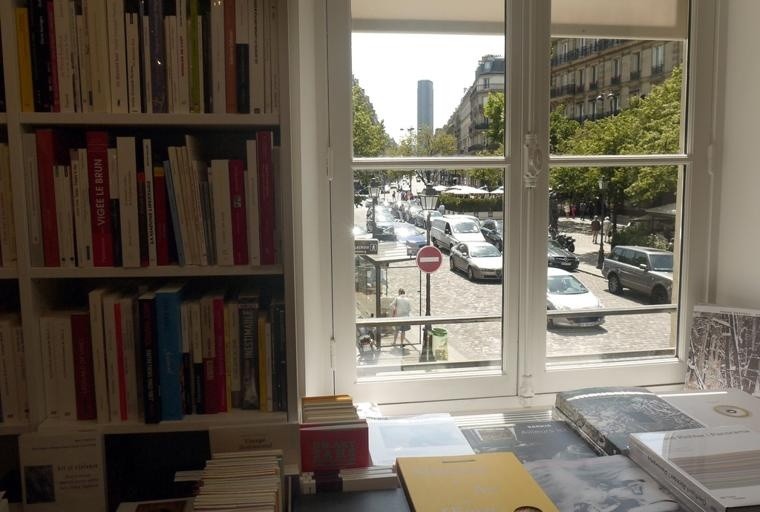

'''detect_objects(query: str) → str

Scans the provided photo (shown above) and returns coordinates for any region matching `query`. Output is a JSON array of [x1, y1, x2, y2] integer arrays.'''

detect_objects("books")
[[1, 280, 286, 422], [19, 421, 303, 511], [16, 1, 280, 116], [2, 129, 281, 270], [683, 304, 759, 398], [299, 386, 759, 510]]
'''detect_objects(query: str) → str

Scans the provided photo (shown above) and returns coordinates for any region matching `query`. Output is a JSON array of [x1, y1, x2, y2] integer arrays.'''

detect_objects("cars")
[[365, 183, 503, 281], [601, 245, 673, 304], [547, 233, 604, 329]]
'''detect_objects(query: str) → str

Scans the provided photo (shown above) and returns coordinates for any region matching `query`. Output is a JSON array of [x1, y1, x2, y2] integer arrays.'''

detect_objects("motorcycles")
[[356, 312, 380, 362]]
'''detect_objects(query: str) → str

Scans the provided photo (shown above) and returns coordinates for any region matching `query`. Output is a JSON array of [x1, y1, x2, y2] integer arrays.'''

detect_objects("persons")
[[399, 205, 405, 223], [389, 288, 411, 346], [392, 190, 407, 201], [438, 202, 445, 215], [367, 208, 372, 218], [591, 215, 612, 243], [565, 199, 587, 221]]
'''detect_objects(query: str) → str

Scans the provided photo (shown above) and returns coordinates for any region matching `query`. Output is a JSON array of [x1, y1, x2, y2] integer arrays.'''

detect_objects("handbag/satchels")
[[392, 307, 397, 317]]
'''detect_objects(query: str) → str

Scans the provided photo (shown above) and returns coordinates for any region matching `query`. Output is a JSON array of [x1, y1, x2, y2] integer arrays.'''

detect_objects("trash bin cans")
[[432, 328, 448, 360]]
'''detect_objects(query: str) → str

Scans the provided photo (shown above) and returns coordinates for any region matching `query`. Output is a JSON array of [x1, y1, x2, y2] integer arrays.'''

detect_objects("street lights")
[[367, 178, 381, 238], [596, 178, 605, 269], [418, 184, 441, 362]]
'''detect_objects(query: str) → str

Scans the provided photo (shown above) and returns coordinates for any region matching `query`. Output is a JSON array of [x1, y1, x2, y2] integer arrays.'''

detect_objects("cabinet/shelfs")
[[1, 2, 317, 438]]
